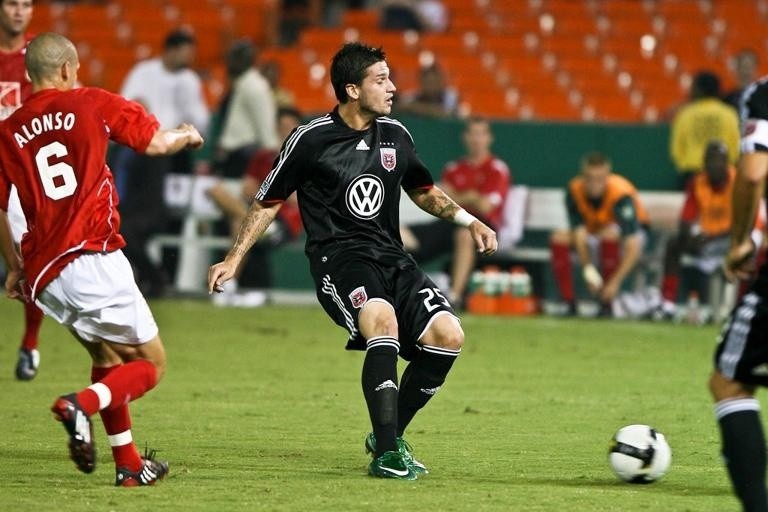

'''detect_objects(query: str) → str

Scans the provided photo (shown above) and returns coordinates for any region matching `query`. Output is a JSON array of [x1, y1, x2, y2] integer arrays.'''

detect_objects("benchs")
[[150, 172, 691, 304]]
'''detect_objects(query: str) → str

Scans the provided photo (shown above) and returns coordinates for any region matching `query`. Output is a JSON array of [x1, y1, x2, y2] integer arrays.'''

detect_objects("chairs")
[[23, 1, 767, 123]]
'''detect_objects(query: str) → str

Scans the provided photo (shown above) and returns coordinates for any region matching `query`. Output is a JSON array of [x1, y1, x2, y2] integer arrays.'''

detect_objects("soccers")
[[610, 425, 673, 484]]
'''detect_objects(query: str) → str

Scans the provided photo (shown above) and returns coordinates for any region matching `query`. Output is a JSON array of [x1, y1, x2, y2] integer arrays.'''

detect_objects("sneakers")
[[209, 274, 271, 309], [540, 296, 604, 319], [14, 345, 40, 380], [363, 430, 430, 477], [367, 450, 420, 481], [640, 299, 717, 324], [114, 455, 169, 487], [51, 391, 96, 474], [445, 286, 464, 311]]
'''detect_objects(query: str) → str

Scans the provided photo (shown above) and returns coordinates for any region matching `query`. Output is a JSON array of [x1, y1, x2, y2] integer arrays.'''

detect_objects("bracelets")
[[453, 206, 478, 228], [582, 263, 604, 288]]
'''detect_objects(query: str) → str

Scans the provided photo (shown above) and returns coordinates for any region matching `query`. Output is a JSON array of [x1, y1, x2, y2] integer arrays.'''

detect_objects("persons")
[[707, 76, 767, 509], [550, 152, 653, 320], [0, 0, 85, 380], [402, 117, 511, 305], [209, 40, 501, 481], [723, 50, 759, 106], [641, 143, 767, 324], [1, 33, 207, 486], [668, 69, 742, 190]]
